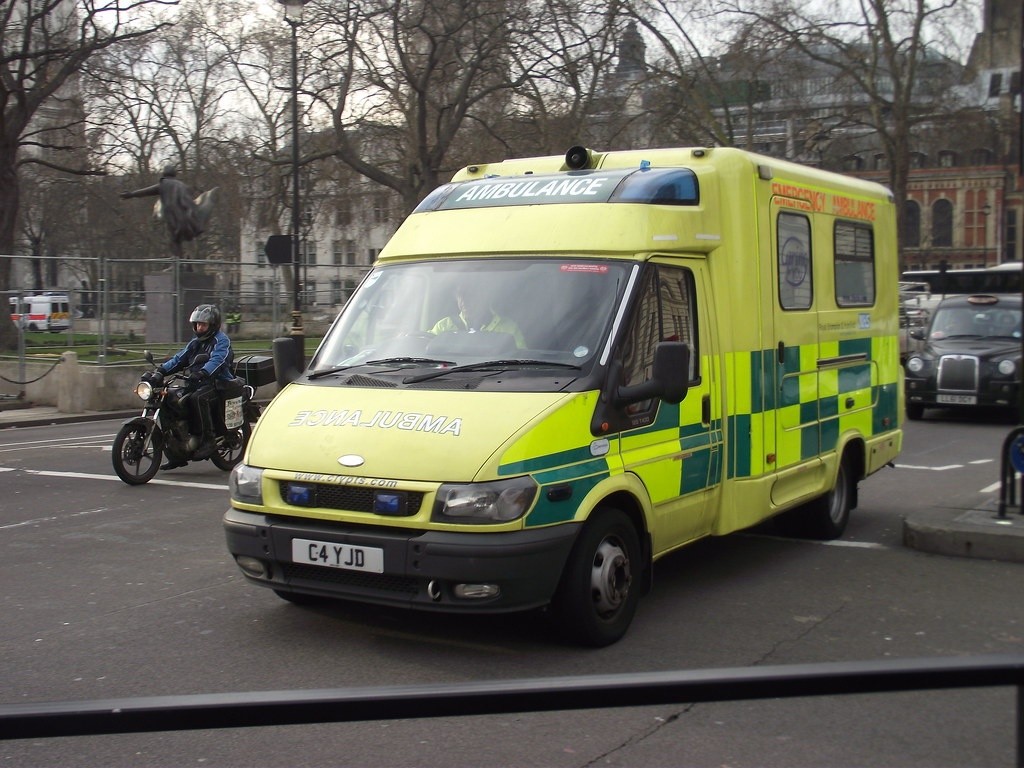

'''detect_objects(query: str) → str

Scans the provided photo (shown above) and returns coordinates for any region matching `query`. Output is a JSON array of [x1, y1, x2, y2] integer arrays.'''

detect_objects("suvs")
[[907, 293, 1024, 422]]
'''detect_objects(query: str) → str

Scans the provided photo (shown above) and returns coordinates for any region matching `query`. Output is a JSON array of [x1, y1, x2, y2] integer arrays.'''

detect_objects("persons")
[[1000, 314, 1016, 337], [73, 305, 95, 319], [427, 282, 527, 350], [153, 305, 236, 471]]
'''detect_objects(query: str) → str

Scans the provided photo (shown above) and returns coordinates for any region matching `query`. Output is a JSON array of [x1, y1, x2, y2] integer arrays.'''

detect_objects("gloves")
[[188, 371, 204, 387]]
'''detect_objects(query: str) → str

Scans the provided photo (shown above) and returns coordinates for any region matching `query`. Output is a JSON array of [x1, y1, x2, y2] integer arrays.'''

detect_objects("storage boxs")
[[232, 354, 277, 387]]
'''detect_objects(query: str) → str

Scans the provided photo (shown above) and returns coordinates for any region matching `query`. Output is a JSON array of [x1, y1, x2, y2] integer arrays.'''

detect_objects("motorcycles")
[[112, 348, 278, 486]]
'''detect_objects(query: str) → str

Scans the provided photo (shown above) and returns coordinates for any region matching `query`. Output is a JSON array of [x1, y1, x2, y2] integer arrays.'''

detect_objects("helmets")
[[189, 305, 222, 343]]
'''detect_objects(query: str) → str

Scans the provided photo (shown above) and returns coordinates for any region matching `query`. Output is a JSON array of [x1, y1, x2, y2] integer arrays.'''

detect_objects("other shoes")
[[160, 460, 188, 470], [193, 435, 218, 461]]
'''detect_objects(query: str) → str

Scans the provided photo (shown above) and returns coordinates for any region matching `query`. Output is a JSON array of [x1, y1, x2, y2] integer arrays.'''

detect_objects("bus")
[[903, 265, 1024, 296]]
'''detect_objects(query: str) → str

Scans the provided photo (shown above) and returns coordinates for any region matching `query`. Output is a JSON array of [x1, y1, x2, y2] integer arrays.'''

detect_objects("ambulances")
[[222, 146, 907, 650]]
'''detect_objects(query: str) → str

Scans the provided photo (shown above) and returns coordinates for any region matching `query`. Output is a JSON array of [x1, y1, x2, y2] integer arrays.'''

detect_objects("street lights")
[[278, 1, 310, 374]]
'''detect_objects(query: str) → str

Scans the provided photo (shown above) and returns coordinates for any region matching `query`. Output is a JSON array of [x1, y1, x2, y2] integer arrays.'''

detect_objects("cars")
[[898, 281, 944, 364]]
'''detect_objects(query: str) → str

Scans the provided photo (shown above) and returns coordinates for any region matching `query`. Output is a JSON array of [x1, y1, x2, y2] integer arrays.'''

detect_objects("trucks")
[[8, 294, 71, 334]]
